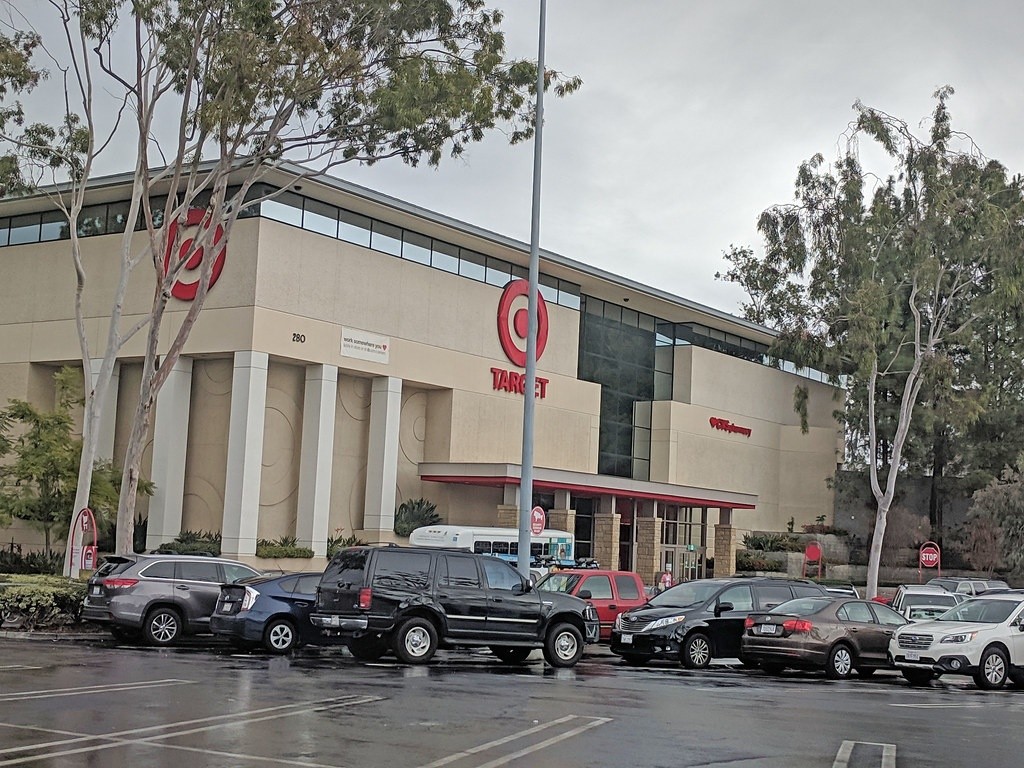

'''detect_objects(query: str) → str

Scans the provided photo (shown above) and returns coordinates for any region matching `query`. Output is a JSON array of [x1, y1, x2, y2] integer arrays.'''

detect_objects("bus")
[[410, 526, 575, 562], [927, 576, 1010, 595]]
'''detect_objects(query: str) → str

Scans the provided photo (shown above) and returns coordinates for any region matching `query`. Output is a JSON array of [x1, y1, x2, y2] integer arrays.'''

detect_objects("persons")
[[660, 569, 675, 590]]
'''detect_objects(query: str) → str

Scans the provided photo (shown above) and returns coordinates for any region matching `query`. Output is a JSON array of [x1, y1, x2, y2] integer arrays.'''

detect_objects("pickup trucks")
[[534, 568, 657, 643]]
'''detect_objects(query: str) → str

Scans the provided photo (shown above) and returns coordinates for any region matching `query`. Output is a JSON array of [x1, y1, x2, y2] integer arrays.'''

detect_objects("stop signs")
[[920, 547, 939, 566]]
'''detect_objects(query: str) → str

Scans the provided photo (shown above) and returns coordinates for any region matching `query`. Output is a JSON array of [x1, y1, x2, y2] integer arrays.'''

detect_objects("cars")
[[952, 592, 974, 603], [210, 570, 323, 657], [740, 595, 944, 680], [901, 604, 963, 622], [821, 582, 862, 599], [611, 574, 830, 670], [888, 588, 1024, 691]]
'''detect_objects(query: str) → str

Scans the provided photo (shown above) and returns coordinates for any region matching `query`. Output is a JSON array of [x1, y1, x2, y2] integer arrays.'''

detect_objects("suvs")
[[78, 551, 266, 648], [309, 542, 600, 668], [889, 586, 960, 616]]
